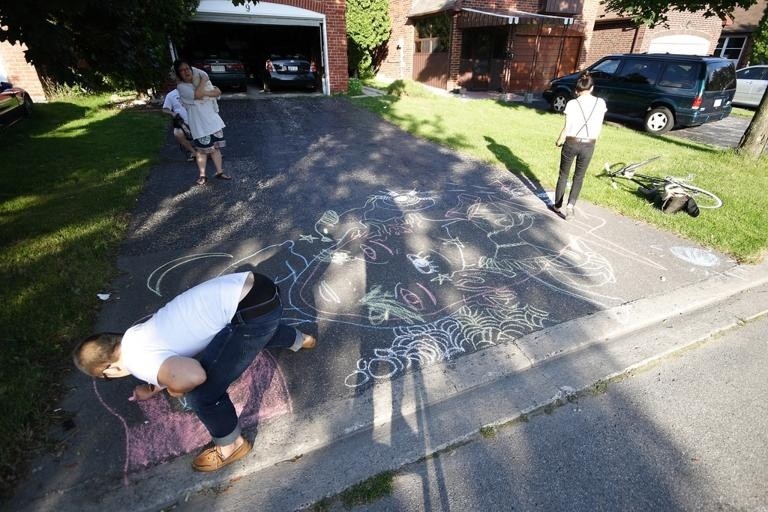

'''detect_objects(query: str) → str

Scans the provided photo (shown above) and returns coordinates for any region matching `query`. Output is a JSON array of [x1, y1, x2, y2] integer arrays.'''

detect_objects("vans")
[[543, 51, 737, 135]]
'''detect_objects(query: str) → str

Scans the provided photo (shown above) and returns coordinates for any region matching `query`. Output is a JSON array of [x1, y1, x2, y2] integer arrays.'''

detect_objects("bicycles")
[[596, 153, 722, 216]]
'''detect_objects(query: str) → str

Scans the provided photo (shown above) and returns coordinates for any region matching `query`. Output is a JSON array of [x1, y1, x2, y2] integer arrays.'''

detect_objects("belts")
[[566, 135, 596, 144], [233, 286, 280, 322]]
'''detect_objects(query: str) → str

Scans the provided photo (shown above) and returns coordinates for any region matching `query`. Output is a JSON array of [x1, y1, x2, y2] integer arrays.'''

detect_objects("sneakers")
[[186, 153, 195, 161], [302, 334, 315, 349], [192, 439, 252, 471]]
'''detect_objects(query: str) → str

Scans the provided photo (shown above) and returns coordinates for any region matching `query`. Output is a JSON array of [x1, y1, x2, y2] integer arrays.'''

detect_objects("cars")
[[0, 81, 34, 138], [731, 65, 768, 109], [193, 50, 248, 90], [262, 50, 319, 93]]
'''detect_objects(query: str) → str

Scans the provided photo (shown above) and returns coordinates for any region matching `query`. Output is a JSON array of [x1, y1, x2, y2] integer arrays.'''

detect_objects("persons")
[[190, 65, 220, 112], [72, 270, 316, 474], [163, 87, 202, 163], [175, 58, 232, 185], [553, 70, 608, 220]]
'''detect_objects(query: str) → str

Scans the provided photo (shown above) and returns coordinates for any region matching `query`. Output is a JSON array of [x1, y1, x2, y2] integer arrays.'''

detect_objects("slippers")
[[550, 204, 560, 213], [565, 207, 575, 222], [196, 176, 208, 185], [215, 172, 231, 180]]
